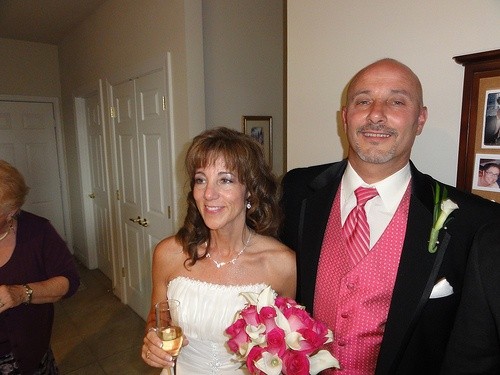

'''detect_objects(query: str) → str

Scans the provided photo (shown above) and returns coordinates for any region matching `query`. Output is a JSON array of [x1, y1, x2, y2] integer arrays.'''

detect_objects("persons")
[[278, 58, 500, 375], [0, 159, 80, 375], [140, 127, 297, 375], [478, 164, 500, 189]]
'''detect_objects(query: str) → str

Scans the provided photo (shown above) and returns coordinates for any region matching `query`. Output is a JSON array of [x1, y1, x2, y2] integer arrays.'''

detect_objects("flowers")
[[225, 285, 340, 375], [428, 181, 458, 254]]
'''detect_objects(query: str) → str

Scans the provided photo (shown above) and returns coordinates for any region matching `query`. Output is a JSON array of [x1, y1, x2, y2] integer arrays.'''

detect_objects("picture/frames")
[[452, 48, 500, 205], [243, 116, 274, 171]]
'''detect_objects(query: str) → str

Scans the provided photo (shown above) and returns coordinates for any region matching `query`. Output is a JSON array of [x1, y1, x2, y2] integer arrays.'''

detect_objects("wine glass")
[[155, 300, 184, 375]]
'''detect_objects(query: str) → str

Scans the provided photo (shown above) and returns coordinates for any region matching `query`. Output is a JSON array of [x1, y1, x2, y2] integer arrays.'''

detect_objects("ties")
[[342, 186, 378, 268]]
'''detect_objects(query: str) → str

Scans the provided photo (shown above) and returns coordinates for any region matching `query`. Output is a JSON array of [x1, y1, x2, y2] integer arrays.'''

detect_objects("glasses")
[[485, 171, 499, 177]]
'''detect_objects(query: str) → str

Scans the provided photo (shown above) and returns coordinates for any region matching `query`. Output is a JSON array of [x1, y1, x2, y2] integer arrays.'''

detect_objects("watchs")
[[23, 283, 33, 307]]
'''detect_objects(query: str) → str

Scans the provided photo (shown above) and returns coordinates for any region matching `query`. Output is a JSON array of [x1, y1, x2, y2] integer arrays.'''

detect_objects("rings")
[[145, 351, 151, 359], [0, 302, 3, 307]]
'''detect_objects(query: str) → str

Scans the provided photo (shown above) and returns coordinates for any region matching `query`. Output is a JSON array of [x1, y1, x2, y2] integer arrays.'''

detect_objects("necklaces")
[[0, 219, 12, 240], [204, 230, 253, 268]]
